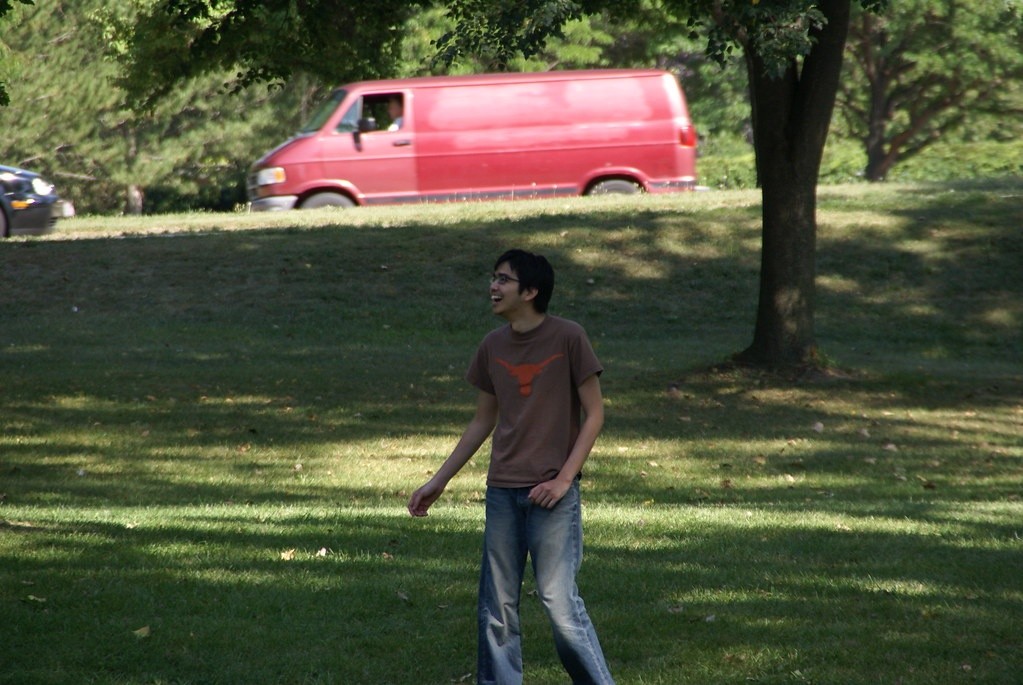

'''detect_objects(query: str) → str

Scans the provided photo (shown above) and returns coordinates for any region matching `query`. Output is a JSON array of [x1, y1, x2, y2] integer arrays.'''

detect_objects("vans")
[[246, 68, 705, 207]]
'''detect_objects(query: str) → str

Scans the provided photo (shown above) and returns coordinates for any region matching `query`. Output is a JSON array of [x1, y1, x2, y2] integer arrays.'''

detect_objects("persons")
[[408, 248, 613, 684], [387, 96, 402, 130]]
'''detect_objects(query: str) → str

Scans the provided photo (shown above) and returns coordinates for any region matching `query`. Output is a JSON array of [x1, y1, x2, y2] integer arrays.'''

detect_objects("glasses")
[[489, 274, 523, 284]]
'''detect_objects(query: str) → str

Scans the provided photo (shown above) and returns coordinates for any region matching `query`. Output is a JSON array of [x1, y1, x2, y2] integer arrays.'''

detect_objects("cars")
[[0, 164, 78, 238]]
